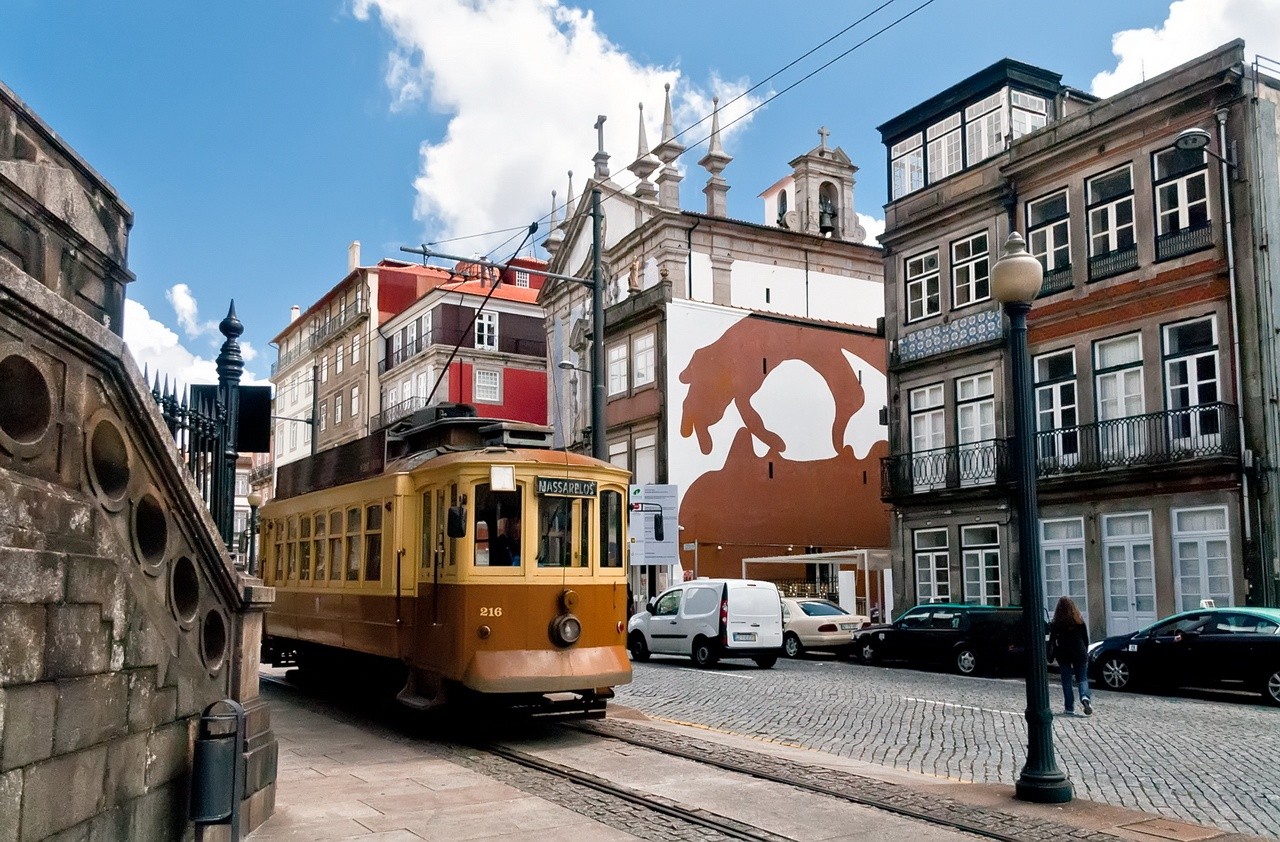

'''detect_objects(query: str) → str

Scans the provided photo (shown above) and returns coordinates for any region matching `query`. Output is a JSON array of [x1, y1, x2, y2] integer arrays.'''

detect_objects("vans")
[[624, 576, 783, 670]]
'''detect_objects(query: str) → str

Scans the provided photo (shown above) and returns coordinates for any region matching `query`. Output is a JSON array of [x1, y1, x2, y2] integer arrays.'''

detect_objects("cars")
[[781, 596, 872, 661], [1086, 605, 1280, 708]]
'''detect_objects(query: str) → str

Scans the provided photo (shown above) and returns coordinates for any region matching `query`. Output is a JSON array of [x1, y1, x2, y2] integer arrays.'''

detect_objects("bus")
[[256, 402, 664, 729]]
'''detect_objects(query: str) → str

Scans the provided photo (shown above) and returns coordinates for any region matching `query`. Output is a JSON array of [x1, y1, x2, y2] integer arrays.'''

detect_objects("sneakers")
[[1063, 709, 1074, 715], [1080, 695, 1093, 715]]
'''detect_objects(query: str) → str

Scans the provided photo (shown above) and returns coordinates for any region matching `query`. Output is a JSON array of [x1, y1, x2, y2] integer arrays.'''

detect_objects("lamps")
[[1171, 127, 1238, 181], [557, 361, 593, 374]]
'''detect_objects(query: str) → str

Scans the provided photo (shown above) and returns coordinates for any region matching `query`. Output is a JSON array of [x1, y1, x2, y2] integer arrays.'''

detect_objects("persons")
[[493, 515, 521, 566], [629, 256, 639, 288], [1049, 597, 1092, 715]]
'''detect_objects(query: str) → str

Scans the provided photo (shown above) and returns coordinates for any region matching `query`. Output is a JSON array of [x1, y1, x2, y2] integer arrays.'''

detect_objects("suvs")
[[853, 603, 1052, 678]]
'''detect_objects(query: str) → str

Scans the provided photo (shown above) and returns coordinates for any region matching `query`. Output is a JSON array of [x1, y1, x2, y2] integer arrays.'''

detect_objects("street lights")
[[988, 231, 1074, 803], [246, 486, 263, 576]]
